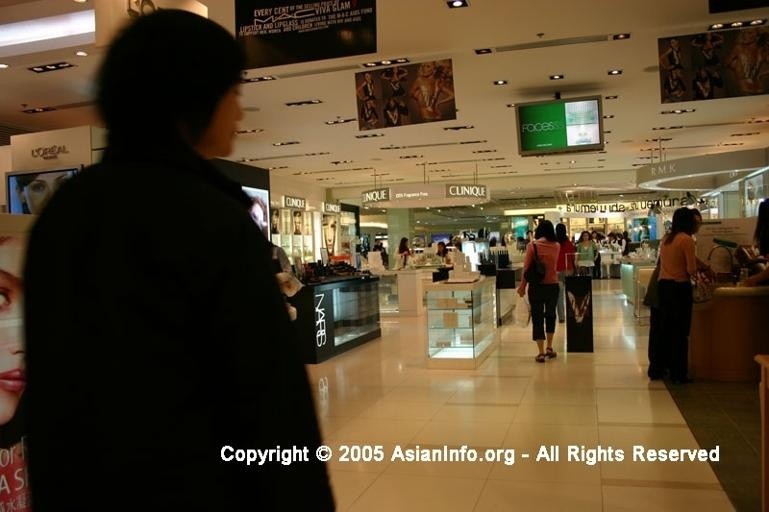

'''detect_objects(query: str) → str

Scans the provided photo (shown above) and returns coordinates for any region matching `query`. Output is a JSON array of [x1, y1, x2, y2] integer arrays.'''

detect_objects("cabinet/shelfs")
[[270, 174, 769, 371]]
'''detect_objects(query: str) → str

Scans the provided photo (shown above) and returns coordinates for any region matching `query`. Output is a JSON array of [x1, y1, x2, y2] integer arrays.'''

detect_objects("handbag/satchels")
[[690, 268, 713, 305], [523, 241, 545, 284]]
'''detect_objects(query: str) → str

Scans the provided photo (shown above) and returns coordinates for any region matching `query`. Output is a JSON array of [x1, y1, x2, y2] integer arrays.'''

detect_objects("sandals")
[[534, 346, 558, 362]]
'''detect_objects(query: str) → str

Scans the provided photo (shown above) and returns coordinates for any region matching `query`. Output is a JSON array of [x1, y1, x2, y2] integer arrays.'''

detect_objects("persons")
[[409, 60, 455, 124], [692, 67, 724, 100], [516, 219, 561, 363], [359, 100, 378, 127], [323, 214, 336, 256], [380, 66, 408, 93], [555, 222, 577, 324], [665, 69, 687, 104], [373, 229, 632, 265], [0, 230, 35, 449], [726, 28, 769, 95], [659, 37, 686, 71], [250, 196, 268, 234], [13, 172, 73, 218], [271, 208, 280, 234], [575, 230, 598, 273], [740, 197, 769, 287], [642, 207, 716, 308], [646, 207, 700, 383], [381, 99, 409, 127], [16, 11, 338, 511], [690, 32, 725, 67], [293, 211, 300, 234], [355, 72, 377, 99]]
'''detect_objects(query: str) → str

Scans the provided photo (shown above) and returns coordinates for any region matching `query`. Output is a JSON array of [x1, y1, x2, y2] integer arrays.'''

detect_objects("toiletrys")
[[290, 256, 372, 283]]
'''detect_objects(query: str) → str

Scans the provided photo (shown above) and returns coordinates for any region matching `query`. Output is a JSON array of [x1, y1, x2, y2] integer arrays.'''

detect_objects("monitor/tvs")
[[514, 94, 604, 156], [5, 163, 84, 215]]
